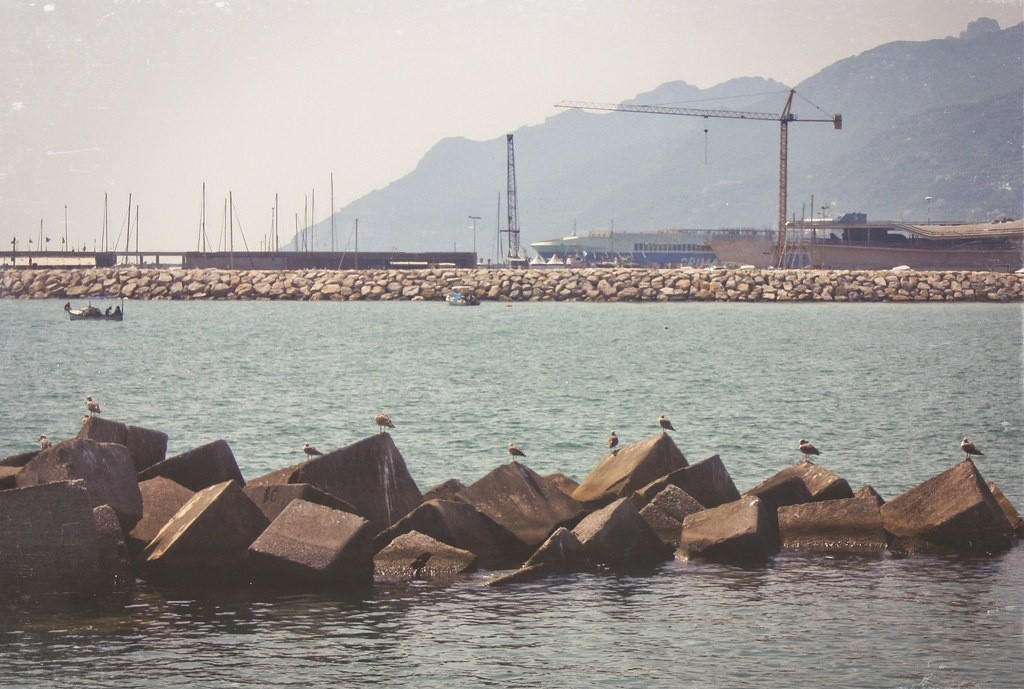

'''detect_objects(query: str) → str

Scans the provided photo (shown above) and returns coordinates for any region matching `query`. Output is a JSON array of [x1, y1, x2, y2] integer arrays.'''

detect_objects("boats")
[[446, 292, 480, 304], [61, 301, 125, 321], [529, 221, 841, 268]]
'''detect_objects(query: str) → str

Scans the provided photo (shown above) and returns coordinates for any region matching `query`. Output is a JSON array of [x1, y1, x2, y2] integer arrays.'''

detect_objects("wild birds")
[[961, 437, 984, 462], [508, 441, 527, 461], [660, 415, 677, 433], [86, 395, 102, 417], [607, 429, 618, 452], [375, 412, 396, 433], [37, 434, 53, 449], [304, 442, 324, 460], [799, 439, 823, 462]]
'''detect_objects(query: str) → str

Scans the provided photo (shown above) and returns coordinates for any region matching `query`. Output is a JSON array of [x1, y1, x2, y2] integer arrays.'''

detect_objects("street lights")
[[468, 215, 482, 252]]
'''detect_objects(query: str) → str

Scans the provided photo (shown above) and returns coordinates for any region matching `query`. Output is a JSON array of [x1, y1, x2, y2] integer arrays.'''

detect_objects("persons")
[[64, 301, 71, 311], [105, 306, 121, 316]]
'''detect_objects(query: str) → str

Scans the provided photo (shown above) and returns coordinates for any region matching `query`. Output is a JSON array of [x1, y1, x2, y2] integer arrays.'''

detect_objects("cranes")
[[551, 87, 844, 270]]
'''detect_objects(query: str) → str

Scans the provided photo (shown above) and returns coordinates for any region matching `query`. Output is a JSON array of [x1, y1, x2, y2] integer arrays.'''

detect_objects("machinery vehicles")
[[502, 130, 530, 269]]
